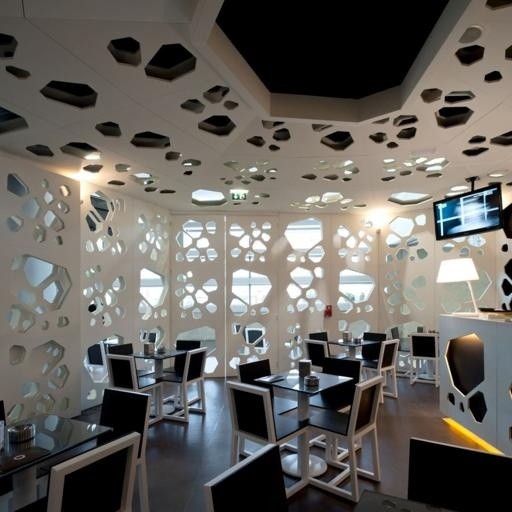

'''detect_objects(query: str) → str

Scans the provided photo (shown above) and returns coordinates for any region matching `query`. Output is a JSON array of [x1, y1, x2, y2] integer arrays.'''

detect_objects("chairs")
[[308, 357, 364, 464], [362, 332, 387, 360], [97, 386, 154, 512], [162, 340, 203, 406], [408, 331, 441, 388], [402, 433, 512, 512], [309, 332, 333, 356], [361, 339, 401, 406], [390, 325, 410, 378], [108, 342, 156, 377], [312, 376, 386, 502], [201, 441, 290, 512], [8, 429, 142, 512], [301, 338, 333, 360], [140, 331, 157, 344], [85, 339, 106, 375], [232, 325, 300, 356], [162, 346, 211, 423], [106, 352, 162, 425], [234, 357, 299, 458], [222, 379, 311, 491]]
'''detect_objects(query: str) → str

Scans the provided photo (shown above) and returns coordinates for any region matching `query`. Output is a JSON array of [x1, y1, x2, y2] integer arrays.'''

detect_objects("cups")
[[298, 359, 312, 378], [341, 330, 353, 344]]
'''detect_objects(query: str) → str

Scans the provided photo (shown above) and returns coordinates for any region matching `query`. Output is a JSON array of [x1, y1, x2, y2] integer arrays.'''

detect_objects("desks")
[[348, 488, 450, 512], [327, 337, 379, 360], [254, 367, 354, 480], [124, 349, 188, 418], [0, 412, 118, 512]]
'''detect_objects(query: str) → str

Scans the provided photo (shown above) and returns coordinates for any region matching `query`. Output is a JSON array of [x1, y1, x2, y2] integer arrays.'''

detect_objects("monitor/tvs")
[[433, 182, 503, 240]]
